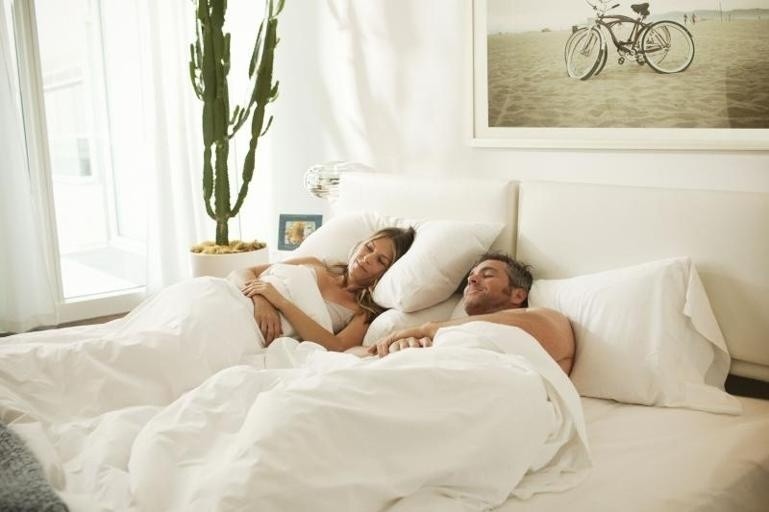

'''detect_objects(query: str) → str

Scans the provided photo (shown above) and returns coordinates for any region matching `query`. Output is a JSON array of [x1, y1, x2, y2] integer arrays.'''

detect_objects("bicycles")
[[564, 0, 696, 81]]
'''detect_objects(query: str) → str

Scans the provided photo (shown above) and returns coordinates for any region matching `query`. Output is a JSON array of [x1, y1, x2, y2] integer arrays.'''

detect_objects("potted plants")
[[188, 0, 285, 282]]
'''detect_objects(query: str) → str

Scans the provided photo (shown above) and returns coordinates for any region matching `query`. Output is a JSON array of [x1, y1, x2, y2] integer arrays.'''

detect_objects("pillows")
[[302, 209, 506, 310], [449, 257, 744, 415], [361, 291, 464, 353]]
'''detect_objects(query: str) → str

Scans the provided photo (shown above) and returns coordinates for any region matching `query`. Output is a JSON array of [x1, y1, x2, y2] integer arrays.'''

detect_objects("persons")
[[683, 12, 696, 24], [366, 251, 576, 376], [227, 226, 417, 352]]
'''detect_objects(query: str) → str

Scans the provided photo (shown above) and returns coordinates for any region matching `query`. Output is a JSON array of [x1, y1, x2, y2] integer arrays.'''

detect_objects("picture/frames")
[[473, 0, 769, 151], [278, 214, 322, 250]]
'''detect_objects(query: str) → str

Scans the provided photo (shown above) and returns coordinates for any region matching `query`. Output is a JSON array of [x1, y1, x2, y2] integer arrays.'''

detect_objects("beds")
[[0, 175, 768, 511]]
